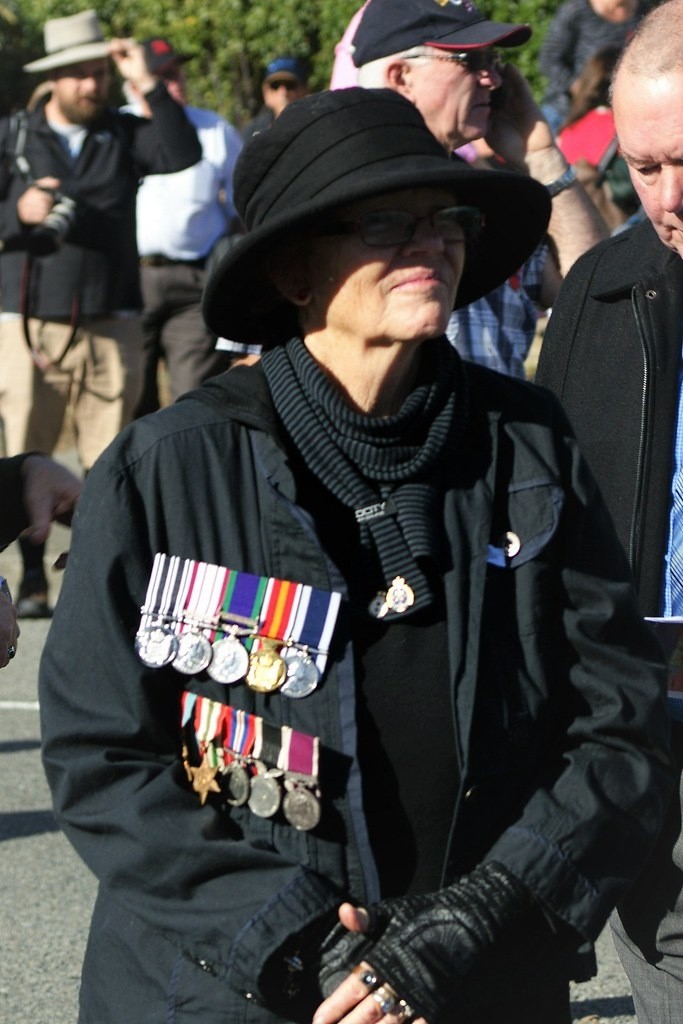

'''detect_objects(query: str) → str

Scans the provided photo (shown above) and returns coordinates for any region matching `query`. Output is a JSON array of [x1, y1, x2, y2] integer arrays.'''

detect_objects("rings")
[[8, 646, 16, 660]]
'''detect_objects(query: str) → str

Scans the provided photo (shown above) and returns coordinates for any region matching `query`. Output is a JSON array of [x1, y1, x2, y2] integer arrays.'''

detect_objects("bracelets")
[[546, 162, 578, 197]]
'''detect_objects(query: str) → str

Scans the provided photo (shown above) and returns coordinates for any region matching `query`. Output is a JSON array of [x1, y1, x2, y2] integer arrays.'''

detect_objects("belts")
[[140, 255, 200, 267]]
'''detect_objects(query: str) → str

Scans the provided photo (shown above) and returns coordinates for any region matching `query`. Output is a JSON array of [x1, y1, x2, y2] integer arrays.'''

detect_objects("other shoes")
[[14, 577, 48, 617]]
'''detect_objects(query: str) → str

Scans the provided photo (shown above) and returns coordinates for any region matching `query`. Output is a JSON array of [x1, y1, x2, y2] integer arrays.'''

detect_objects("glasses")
[[285, 205, 486, 248], [401, 51, 500, 73], [270, 82, 298, 91]]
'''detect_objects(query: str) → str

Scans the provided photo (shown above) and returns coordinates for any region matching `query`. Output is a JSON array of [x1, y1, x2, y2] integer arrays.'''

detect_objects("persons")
[[115, 34, 246, 428], [552, 45, 626, 171], [353, 0, 613, 378], [0, 9, 204, 621], [531, 2, 682, 1023], [241, 56, 307, 140], [536, 0, 644, 134], [0, 449, 85, 669], [34, 88, 665, 1023]]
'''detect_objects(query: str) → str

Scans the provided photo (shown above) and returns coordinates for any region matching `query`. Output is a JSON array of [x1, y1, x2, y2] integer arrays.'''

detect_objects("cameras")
[[29, 190, 90, 259]]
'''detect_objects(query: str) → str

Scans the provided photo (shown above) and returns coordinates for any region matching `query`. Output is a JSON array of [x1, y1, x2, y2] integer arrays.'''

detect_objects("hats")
[[23, 9, 124, 73], [145, 38, 198, 73], [264, 58, 306, 79], [201, 85, 551, 343], [352, 0, 532, 67]]
